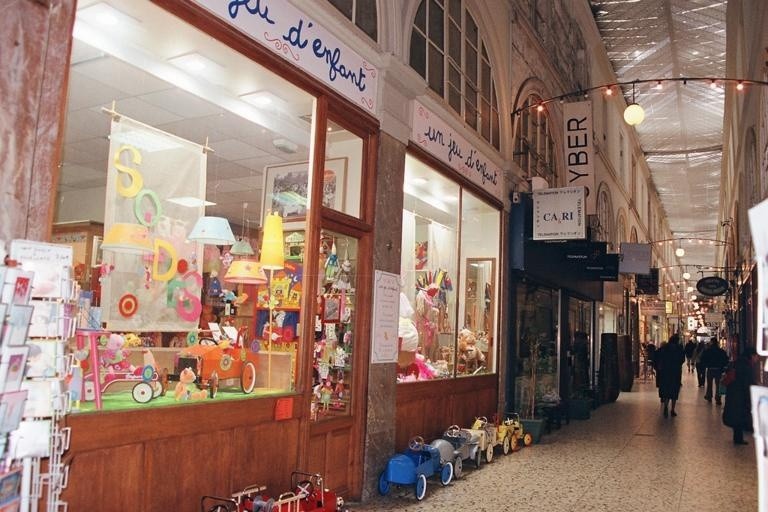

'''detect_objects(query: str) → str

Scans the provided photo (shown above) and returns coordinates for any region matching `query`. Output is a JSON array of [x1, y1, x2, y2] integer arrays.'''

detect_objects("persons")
[[722, 346, 763, 445], [569, 330, 588, 399], [647, 332, 729, 418]]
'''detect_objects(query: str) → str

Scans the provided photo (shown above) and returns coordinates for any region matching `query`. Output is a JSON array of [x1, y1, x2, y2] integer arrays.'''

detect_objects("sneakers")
[[664, 408, 677, 417], [704, 395, 721, 405], [734, 440, 749, 444]]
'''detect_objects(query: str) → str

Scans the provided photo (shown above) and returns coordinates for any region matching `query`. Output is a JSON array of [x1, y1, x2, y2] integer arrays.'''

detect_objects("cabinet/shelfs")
[[250, 234, 306, 392]]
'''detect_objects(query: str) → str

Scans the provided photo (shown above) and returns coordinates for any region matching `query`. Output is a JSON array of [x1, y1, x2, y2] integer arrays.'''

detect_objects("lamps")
[[231, 204, 256, 257], [189, 162, 237, 246], [224, 261, 267, 286], [510, 78, 768, 126], [260, 214, 285, 392], [676, 239, 705, 327], [99, 223, 152, 256]]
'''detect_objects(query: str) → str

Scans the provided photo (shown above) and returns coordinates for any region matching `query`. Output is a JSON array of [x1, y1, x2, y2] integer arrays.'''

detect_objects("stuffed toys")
[[311, 236, 355, 421], [397, 284, 489, 378], [81, 326, 256, 404]]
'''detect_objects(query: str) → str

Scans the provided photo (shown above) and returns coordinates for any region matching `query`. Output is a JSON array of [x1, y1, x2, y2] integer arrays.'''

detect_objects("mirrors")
[[463, 257, 497, 371]]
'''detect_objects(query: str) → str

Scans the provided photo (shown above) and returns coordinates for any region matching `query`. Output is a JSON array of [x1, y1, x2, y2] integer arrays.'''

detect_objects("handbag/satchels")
[[721, 367, 736, 386]]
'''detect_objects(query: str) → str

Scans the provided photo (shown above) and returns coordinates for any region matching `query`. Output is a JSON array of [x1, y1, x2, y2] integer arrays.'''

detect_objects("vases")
[[519, 415, 548, 444]]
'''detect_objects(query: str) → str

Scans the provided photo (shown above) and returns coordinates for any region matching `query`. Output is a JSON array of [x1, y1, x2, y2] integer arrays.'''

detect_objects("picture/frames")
[[259, 156, 348, 228]]
